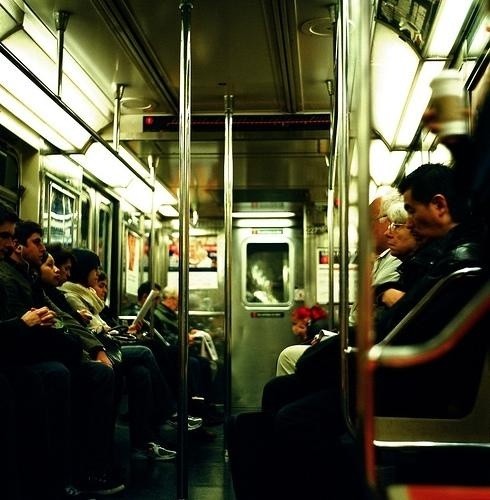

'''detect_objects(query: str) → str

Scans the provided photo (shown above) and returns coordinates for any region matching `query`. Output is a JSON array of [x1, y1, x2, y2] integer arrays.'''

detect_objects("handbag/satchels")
[[108, 324, 143, 346], [80, 328, 123, 367]]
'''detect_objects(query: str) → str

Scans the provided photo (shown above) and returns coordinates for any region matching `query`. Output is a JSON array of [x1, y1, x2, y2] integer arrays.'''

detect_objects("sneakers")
[[72, 470, 125, 495], [131, 442, 177, 462], [47, 488, 99, 500], [161, 414, 204, 432]]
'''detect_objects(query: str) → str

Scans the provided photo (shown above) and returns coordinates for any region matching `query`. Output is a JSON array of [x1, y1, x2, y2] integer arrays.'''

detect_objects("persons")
[[0, 199, 227, 500], [223, 158, 489, 499]]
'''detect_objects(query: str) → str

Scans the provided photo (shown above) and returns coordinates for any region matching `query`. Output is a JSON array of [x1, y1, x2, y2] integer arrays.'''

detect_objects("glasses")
[[388, 220, 408, 233]]
